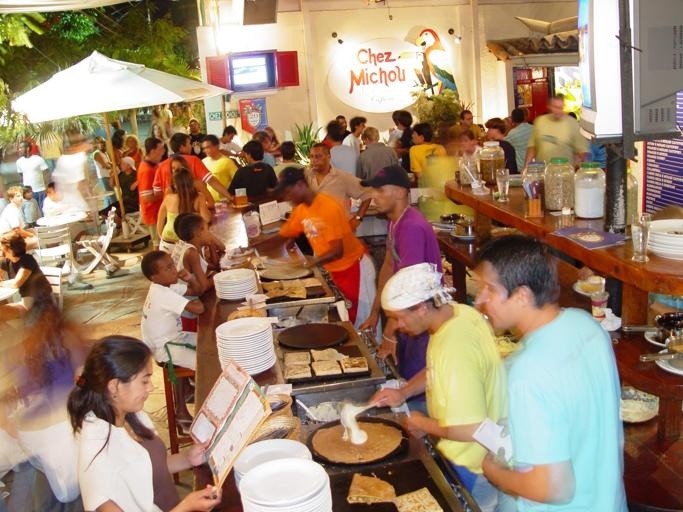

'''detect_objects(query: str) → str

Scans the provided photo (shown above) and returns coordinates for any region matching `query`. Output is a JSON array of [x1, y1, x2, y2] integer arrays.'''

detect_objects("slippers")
[[175, 409, 194, 432]]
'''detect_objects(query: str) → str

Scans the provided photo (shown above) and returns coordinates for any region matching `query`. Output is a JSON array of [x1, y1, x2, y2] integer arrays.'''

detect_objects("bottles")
[[521, 158, 638, 225], [459, 157, 479, 185], [478, 140, 505, 184]]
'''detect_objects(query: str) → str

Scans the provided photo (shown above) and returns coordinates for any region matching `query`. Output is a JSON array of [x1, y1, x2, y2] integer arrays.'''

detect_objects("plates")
[[216, 302, 244, 316], [214, 315, 276, 379], [230, 439, 333, 512], [649, 218, 683, 261], [432, 217, 516, 241], [214, 267, 258, 300], [642, 326, 682, 376]]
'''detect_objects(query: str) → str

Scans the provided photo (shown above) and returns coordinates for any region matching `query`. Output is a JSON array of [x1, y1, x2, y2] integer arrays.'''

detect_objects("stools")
[[155, 360, 195, 485]]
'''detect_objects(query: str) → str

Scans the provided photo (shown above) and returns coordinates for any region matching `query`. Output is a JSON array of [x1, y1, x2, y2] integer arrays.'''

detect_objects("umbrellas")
[[0, 49, 232, 239]]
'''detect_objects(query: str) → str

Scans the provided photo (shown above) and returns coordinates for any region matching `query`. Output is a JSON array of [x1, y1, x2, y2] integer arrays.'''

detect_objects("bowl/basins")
[[656, 313, 682, 342], [228, 246, 255, 261], [573, 278, 606, 298]]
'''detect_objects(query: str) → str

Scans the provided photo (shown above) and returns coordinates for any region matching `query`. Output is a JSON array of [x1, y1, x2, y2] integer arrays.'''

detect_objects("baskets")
[[227, 308, 267, 322], [248, 392, 301, 446]]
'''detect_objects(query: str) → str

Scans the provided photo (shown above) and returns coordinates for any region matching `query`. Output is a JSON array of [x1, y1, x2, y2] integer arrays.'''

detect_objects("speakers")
[[243, 0, 278, 25]]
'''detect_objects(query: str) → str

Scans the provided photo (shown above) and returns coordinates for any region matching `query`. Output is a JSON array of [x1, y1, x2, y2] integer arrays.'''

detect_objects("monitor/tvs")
[[229, 53, 276, 92]]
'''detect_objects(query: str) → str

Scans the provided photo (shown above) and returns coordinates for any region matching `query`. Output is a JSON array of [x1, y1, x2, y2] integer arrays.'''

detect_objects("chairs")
[[125, 205, 150, 237], [85, 196, 103, 234], [34, 223, 81, 284], [76, 207, 122, 274], [37, 265, 63, 313]]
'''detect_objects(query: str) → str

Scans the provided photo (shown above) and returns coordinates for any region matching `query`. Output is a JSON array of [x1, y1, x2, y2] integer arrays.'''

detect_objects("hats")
[[121, 156, 137, 170], [359, 163, 411, 188], [270, 166, 304, 194]]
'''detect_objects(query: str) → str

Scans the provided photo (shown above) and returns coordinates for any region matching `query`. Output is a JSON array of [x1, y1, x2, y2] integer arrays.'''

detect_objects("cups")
[[495, 169, 510, 202], [214, 197, 228, 213], [631, 212, 650, 262], [591, 291, 609, 323], [471, 173, 484, 191]]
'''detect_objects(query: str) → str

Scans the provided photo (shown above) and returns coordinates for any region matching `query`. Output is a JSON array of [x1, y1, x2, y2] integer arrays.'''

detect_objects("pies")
[[262, 277, 322, 299], [393, 487, 444, 512], [283, 347, 369, 379], [346, 472, 396, 504]]
